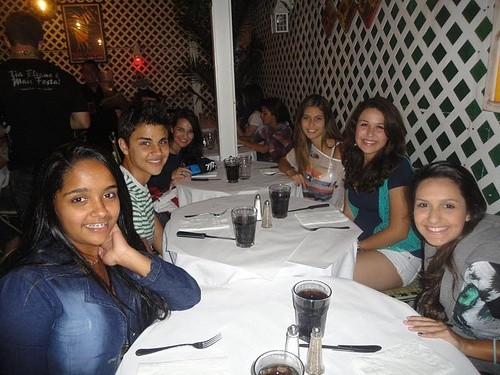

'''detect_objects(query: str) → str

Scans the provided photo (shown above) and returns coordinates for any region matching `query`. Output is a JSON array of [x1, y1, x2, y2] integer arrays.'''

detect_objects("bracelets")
[[357, 243, 361, 252], [492, 337, 497, 364], [284, 167, 294, 175]]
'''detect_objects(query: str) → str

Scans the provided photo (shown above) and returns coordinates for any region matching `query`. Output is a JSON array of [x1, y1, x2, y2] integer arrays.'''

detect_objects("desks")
[[162, 193, 363, 279], [115, 276, 479, 375], [176, 143, 304, 208]]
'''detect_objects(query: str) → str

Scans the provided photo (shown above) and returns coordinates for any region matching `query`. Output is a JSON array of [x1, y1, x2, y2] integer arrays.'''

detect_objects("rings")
[[180, 172, 183, 175]]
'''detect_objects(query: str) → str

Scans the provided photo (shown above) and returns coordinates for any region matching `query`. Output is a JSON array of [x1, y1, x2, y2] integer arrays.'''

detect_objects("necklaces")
[[10, 51, 36, 57], [87, 258, 100, 264]]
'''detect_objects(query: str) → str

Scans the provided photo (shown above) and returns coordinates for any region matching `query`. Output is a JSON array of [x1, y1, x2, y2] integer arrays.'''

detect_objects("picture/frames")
[[60, 2, 108, 65]]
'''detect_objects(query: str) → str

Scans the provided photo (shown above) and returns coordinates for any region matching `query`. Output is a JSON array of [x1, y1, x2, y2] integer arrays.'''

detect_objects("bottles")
[[254, 194, 262, 221], [305, 325, 325, 375], [285, 325, 299, 362], [261, 200, 272, 228]]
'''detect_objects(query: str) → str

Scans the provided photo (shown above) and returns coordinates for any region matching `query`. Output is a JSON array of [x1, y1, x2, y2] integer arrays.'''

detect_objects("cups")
[[293, 280, 332, 343], [231, 206, 257, 248], [204, 132, 216, 149], [253, 350, 305, 375], [238, 156, 252, 180], [268, 183, 291, 219], [224, 157, 239, 183]]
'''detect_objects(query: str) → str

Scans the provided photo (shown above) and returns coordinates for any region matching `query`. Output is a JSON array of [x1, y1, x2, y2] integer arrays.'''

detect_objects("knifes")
[[299, 343, 382, 353], [288, 203, 329, 212], [258, 165, 278, 169], [177, 231, 235, 240], [191, 178, 221, 181]]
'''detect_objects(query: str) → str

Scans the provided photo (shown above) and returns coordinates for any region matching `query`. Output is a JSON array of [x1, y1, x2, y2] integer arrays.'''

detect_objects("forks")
[[135, 332, 222, 356], [184, 208, 228, 217], [300, 225, 350, 231]]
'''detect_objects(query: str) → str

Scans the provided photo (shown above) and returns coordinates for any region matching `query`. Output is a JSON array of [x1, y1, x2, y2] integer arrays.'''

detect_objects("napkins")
[[178, 211, 229, 231], [295, 210, 349, 226]]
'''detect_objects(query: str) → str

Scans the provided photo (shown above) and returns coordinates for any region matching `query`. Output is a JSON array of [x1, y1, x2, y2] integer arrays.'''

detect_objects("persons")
[[0, 12, 91, 237], [0, 60, 202, 375], [235, 84, 500, 375]]
